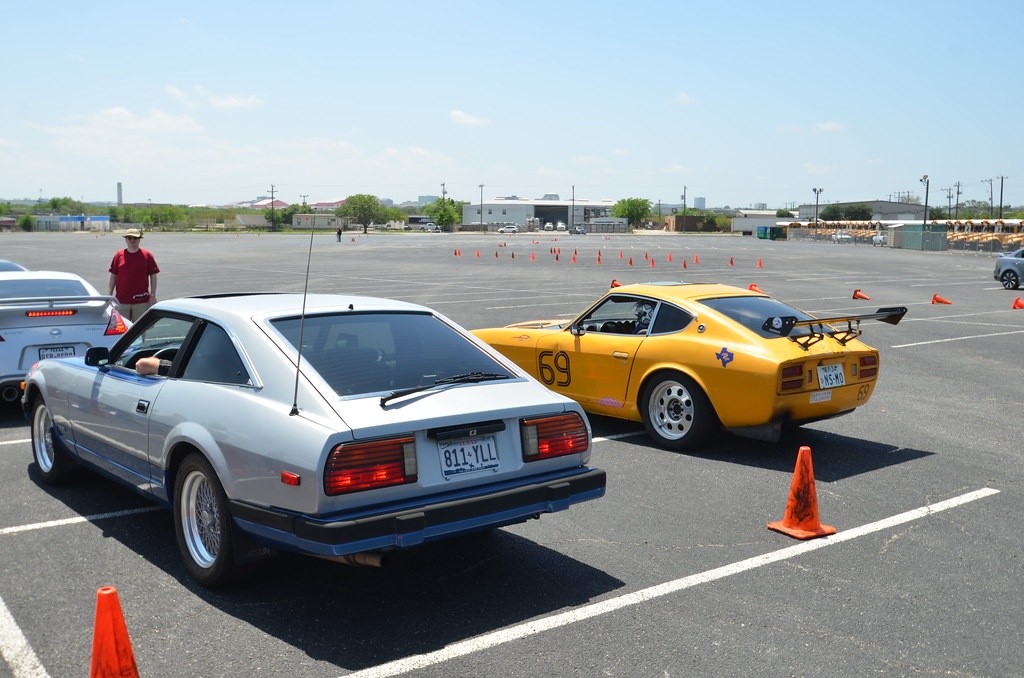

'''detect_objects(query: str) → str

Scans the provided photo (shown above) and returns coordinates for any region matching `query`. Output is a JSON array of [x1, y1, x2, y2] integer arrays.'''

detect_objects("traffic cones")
[[91, 587, 139, 677], [453, 239, 734, 270], [611, 281, 622, 288], [748, 283, 765, 293], [931, 292, 952, 306], [766, 445, 838, 540], [1010, 297, 1024, 310], [757, 259, 764, 268], [851, 288, 872, 302]]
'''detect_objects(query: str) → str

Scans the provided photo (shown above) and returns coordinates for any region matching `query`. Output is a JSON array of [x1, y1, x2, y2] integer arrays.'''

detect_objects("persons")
[[109, 229, 160, 322], [136, 358, 172, 375], [337, 228, 341, 242]]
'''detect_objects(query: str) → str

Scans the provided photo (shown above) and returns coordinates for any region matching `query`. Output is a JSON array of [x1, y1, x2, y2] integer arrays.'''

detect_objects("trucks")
[[386, 220, 404, 230]]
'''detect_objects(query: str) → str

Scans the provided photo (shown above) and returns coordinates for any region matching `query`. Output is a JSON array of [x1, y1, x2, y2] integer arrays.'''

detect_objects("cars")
[[0, 259, 135, 402], [544, 222, 554, 231], [498, 225, 520, 234], [20, 291, 607, 586], [556, 222, 567, 231], [831, 231, 852, 245], [992, 248, 1024, 290]]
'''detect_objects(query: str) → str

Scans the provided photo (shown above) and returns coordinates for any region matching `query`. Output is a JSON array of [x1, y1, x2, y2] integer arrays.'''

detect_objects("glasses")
[[127, 236, 139, 240]]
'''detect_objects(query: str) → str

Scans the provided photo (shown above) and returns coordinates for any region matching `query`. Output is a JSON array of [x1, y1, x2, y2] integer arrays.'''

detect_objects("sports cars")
[[468, 280, 879, 452]]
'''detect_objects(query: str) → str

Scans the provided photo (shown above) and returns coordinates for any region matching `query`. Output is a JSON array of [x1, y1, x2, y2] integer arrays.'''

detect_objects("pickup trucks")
[[872, 231, 888, 246], [419, 222, 436, 231], [569, 226, 587, 235]]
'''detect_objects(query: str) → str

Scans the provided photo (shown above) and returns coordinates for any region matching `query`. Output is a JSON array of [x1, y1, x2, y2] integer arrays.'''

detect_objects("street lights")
[[921, 175, 929, 226], [812, 187, 824, 235]]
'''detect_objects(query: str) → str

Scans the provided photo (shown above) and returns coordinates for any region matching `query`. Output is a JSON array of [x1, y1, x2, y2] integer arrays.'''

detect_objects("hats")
[[123, 228, 141, 237]]
